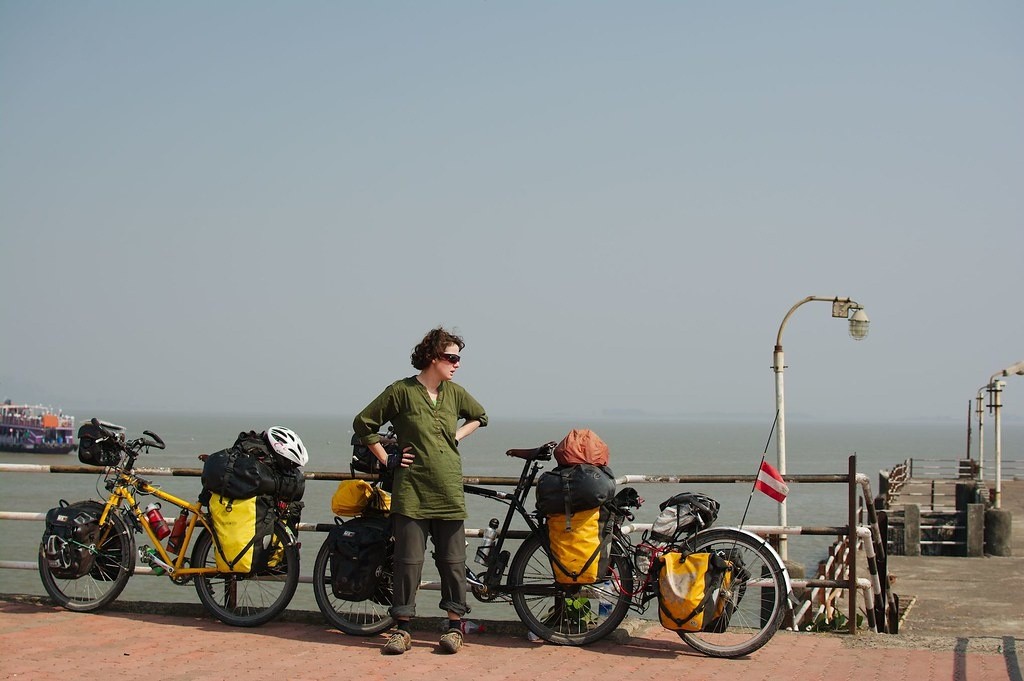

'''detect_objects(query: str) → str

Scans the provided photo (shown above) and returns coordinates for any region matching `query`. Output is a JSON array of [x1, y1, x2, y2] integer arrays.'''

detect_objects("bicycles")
[[510, 483, 802, 659], [312, 429, 637, 646], [36, 417, 302, 628]]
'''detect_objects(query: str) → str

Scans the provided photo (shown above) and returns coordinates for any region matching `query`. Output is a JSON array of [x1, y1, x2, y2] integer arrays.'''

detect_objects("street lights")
[[769, 293, 869, 561], [976, 361, 1024, 509]]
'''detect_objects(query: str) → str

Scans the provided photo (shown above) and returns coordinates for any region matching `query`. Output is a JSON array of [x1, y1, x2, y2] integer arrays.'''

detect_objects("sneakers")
[[383, 628, 411, 653], [440, 628, 464, 653]]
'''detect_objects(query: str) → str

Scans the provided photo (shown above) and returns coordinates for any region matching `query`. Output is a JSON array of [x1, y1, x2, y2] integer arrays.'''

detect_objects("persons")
[[352, 329, 489, 653]]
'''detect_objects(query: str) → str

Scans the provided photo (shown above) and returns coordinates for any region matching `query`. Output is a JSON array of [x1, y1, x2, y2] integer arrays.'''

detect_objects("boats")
[[0, 398, 79, 456]]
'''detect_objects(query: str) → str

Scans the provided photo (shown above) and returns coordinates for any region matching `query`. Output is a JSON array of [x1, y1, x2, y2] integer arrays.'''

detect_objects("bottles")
[[429, 550, 440, 569], [166, 508, 189, 554], [145, 544, 166, 576], [474, 518, 499, 564], [596, 581, 612, 627], [441, 619, 486, 633], [146, 503, 171, 541]]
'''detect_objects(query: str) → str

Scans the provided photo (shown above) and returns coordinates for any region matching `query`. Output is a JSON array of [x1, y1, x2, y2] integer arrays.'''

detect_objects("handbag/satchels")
[[329, 516, 397, 605], [78, 423, 125, 466], [648, 544, 752, 632], [554, 429, 609, 467], [201, 429, 305, 577], [534, 463, 615, 513], [351, 431, 398, 474], [43, 499, 136, 581], [545, 505, 618, 584]]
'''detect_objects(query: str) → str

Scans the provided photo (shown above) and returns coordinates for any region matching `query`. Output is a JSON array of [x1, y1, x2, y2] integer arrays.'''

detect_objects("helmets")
[[660, 491, 719, 535], [268, 426, 309, 470]]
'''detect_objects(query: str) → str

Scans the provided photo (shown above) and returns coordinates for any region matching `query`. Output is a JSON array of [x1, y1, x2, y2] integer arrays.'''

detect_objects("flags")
[[756, 463, 790, 503]]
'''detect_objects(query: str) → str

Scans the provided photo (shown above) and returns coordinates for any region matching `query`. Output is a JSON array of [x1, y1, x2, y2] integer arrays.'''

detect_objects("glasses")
[[428, 352, 460, 364]]
